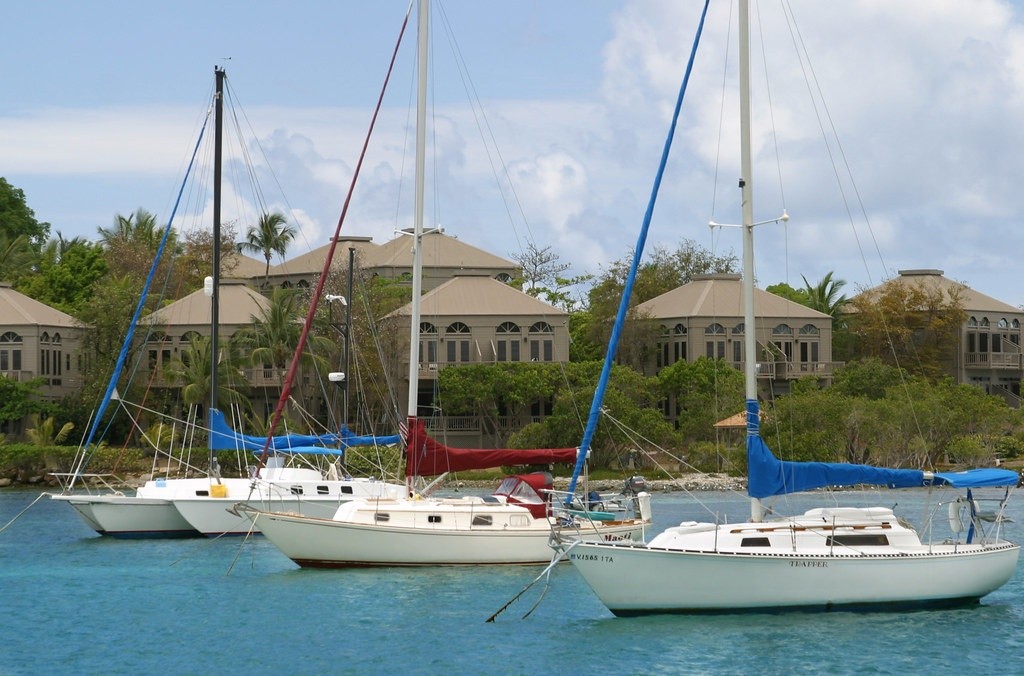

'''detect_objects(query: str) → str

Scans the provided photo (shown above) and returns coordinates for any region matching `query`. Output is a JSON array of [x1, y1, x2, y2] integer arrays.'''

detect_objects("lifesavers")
[[949, 496, 980, 533]]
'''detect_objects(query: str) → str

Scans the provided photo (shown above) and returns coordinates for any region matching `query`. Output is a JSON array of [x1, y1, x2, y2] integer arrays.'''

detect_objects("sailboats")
[[536, 0, 1023, 619], [42, 1, 656, 569]]
